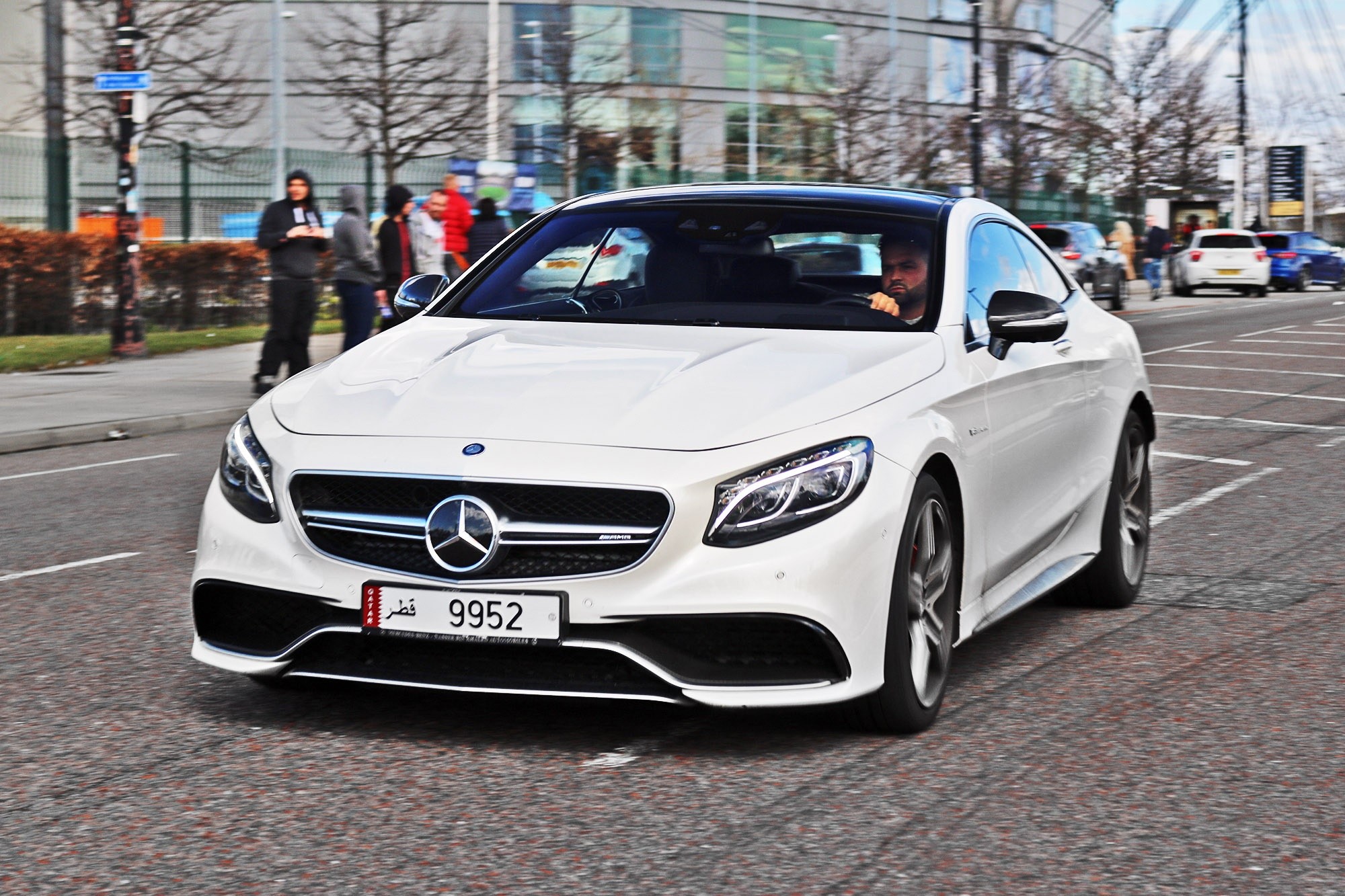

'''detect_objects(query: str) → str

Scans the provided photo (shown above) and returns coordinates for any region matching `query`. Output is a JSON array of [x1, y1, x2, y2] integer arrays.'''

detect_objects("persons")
[[1105, 214, 1268, 302], [250, 170, 508, 396], [865, 228, 931, 325]]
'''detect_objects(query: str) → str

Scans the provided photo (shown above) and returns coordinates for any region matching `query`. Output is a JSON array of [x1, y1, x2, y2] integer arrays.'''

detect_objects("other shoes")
[[1153, 287, 1162, 300], [253, 375, 275, 394]]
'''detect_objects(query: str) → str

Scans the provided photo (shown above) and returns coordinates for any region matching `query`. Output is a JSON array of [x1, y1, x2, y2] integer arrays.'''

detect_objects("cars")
[[1167, 228, 1272, 297], [517, 224, 651, 293], [1257, 230, 1345, 291], [191, 182, 1157, 735]]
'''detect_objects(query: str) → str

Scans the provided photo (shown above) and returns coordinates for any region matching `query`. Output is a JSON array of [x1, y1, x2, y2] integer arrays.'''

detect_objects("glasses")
[[878, 230, 927, 254]]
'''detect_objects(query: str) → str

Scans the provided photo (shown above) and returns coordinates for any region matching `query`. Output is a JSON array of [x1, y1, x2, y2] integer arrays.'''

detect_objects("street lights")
[[821, 34, 848, 180], [1303, 141, 1326, 231], [275, 10, 297, 199], [524, 19, 544, 164], [726, 24, 758, 182]]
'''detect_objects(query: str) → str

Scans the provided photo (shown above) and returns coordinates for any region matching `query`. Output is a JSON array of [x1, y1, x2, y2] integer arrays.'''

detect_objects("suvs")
[[1023, 222, 1121, 293]]
